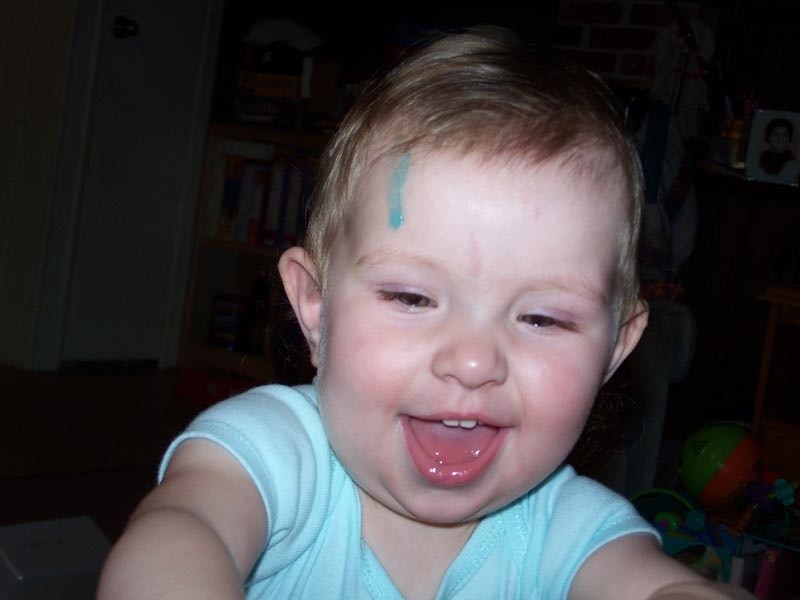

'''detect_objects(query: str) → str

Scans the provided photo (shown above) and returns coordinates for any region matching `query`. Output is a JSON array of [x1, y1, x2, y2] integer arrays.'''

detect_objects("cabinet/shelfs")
[[182, 0, 699, 385]]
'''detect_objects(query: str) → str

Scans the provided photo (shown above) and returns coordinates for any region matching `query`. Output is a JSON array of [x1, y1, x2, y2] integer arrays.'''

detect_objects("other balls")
[[682, 422, 759, 507]]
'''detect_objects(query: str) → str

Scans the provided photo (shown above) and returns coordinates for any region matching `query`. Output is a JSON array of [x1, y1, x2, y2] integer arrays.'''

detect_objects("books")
[[200, 137, 320, 247]]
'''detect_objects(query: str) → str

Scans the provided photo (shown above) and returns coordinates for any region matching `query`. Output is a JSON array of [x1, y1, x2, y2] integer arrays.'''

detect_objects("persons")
[[98, 35, 754, 600], [756, 117, 799, 184]]
